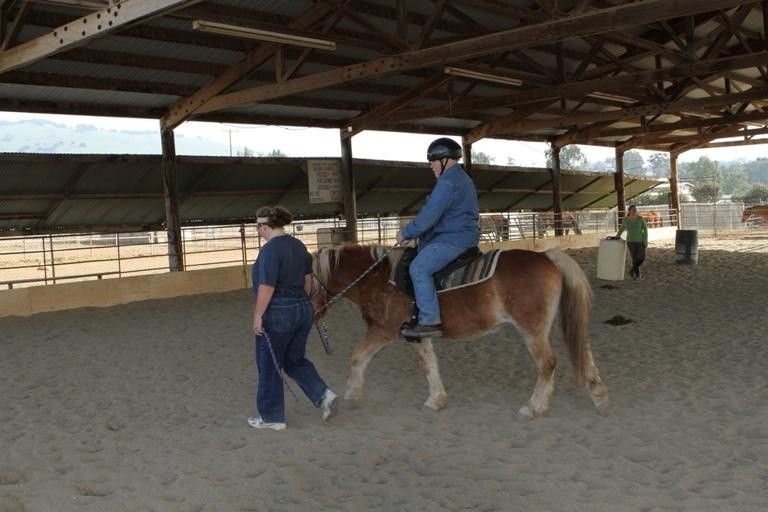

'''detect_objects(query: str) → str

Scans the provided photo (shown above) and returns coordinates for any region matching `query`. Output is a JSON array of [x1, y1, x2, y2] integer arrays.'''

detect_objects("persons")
[[394, 137, 481, 338], [615, 204, 648, 282], [245, 204, 341, 430]]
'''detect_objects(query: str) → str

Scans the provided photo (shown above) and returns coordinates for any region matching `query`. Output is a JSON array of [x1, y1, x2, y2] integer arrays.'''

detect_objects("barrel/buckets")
[[596, 236, 628, 280], [674, 230, 699, 265]]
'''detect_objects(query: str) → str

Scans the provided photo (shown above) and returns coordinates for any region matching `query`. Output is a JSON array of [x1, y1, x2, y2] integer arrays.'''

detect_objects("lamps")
[[192, 20, 336, 52], [585, 91, 641, 104], [444, 67, 523, 87]]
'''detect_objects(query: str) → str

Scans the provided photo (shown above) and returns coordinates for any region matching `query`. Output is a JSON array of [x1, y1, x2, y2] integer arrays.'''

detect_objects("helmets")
[[426, 138, 461, 162]]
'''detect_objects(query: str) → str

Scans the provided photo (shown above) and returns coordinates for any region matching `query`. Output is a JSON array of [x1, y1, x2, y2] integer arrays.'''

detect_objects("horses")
[[653, 210, 664, 227], [304, 243, 610, 422], [640, 210, 656, 227], [741, 204, 768, 226], [480, 213, 510, 241], [535, 212, 582, 237]]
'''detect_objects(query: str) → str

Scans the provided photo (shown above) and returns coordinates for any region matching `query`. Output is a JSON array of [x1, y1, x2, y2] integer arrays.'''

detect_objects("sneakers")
[[319, 389, 339, 422], [633, 275, 640, 281], [400, 324, 441, 338], [247, 417, 286, 431], [628, 270, 634, 278]]
